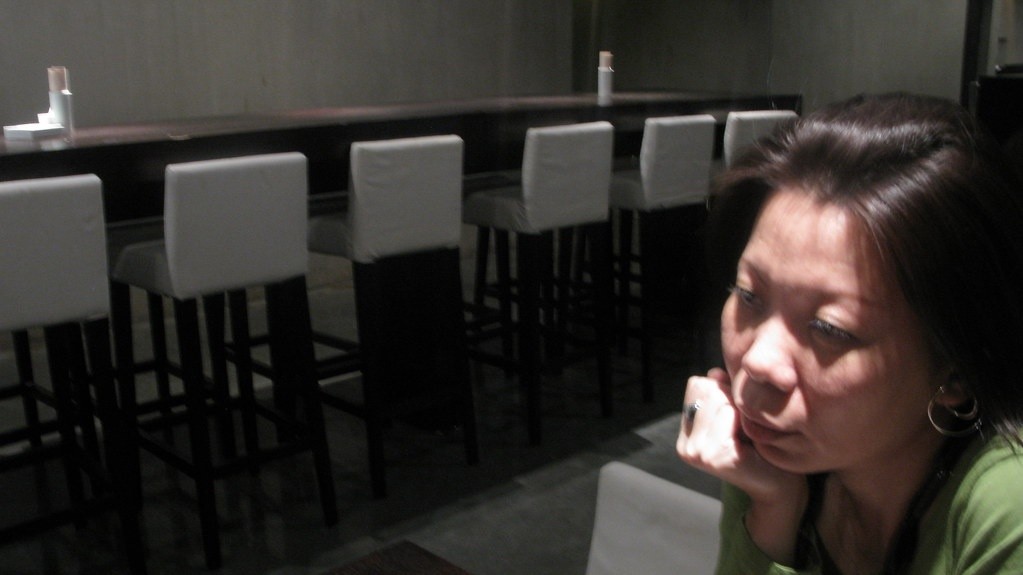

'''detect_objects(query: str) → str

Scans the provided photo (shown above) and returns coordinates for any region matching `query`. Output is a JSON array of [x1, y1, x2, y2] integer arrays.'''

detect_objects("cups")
[[597, 67, 615, 106], [49, 90, 72, 138], [37, 113, 52, 124]]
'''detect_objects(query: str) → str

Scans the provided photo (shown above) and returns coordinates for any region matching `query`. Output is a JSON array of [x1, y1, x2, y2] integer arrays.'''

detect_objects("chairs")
[[588, 461, 725, 574]]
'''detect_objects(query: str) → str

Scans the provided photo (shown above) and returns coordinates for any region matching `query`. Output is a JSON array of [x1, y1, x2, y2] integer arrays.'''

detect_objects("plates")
[[2, 123, 66, 140]]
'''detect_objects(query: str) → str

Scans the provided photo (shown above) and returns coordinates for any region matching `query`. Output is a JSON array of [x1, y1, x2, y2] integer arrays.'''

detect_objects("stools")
[[575, 113, 719, 406], [0, 173, 149, 575], [463, 120, 616, 444], [107, 151, 340, 575], [289, 132, 479, 494], [685, 107, 797, 364]]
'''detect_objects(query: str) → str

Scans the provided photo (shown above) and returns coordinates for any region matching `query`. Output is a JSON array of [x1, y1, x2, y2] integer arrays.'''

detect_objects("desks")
[[1, 83, 804, 516]]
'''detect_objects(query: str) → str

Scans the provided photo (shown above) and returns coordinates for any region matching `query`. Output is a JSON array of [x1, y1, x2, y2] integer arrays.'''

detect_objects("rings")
[[681, 401, 702, 421]]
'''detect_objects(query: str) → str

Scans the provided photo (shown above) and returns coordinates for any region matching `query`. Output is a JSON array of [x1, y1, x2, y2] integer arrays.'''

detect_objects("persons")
[[674, 91, 1023, 575]]
